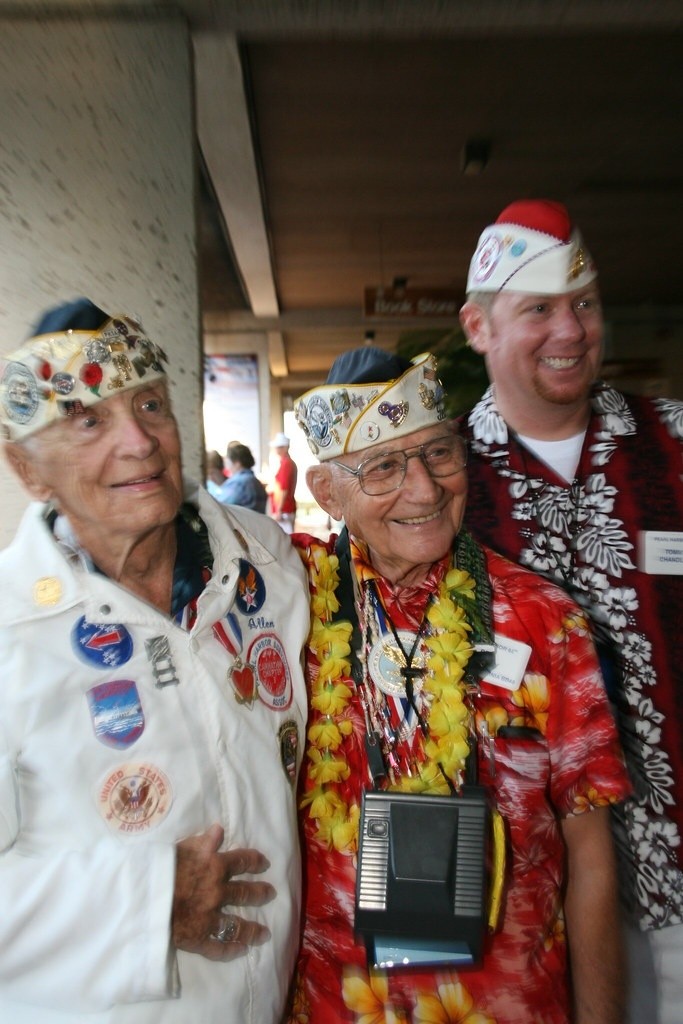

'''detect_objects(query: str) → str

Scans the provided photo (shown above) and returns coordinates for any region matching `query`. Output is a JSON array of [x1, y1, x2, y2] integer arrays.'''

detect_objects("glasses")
[[330, 436, 468, 496]]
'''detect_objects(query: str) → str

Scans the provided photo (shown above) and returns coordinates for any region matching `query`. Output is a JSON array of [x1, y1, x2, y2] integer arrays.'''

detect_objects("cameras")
[[353, 789, 495, 973]]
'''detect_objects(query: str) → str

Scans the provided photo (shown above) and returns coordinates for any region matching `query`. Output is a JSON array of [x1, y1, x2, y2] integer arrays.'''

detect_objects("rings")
[[212, 914, 238, 946]]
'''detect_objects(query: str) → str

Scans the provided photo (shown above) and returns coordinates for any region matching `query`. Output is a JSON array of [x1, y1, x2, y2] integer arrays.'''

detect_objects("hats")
[[269, 435, 290, 447], [295, 348, 451, 462], [0, 297, 169, 443], [465, 200, 598, 294]]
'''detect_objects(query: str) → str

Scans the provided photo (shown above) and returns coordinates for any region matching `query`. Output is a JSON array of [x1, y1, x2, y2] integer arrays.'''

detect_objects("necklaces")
[[361, 581, 447, 745], [508, 407, 595, 597]]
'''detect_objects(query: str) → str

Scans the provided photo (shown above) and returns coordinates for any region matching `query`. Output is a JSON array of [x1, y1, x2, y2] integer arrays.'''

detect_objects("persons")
[[459, 199, 683, 1024], [0, 296, 314, 1024], [289, 349, 634, 1024]]
